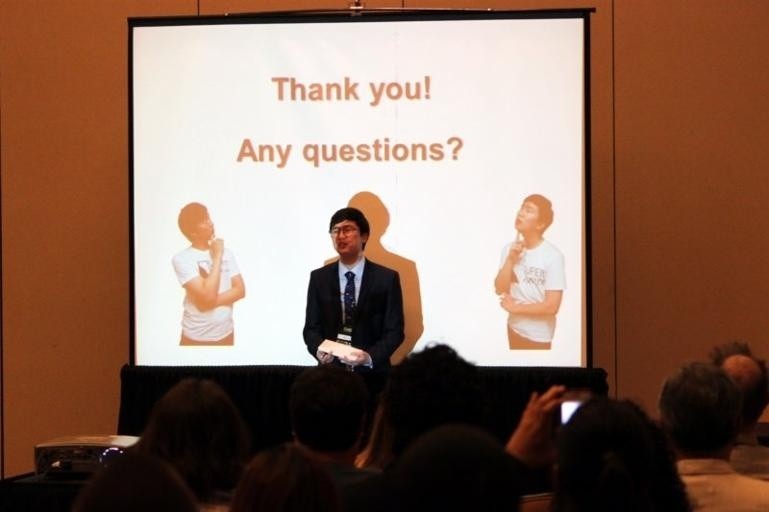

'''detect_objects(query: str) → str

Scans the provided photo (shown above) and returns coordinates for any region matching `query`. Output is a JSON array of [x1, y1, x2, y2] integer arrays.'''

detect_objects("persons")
[[76, 343, 769, 511], [172, 201, 246, 348], [303, 206, 405, 372], [494, 193, 565, 350]]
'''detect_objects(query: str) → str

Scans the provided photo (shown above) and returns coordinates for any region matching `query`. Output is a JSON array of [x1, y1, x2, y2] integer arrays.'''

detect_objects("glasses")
[[330, 228, 357, 236]]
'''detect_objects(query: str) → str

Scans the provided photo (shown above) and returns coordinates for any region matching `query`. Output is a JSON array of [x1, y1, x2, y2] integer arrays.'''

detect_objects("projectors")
[[34, 434, 143, 484]]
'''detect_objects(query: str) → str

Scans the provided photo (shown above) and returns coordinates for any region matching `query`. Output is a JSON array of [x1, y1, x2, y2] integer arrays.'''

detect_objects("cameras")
[[553, 397, 587, 427]]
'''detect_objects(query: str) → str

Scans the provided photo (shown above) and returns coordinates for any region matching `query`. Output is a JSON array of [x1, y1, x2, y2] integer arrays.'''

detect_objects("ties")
[[344, 272, 356, 328]]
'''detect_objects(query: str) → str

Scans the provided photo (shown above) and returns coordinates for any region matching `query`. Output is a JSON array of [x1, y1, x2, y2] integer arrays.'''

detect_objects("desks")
[[0, 473, 89, 512]]
